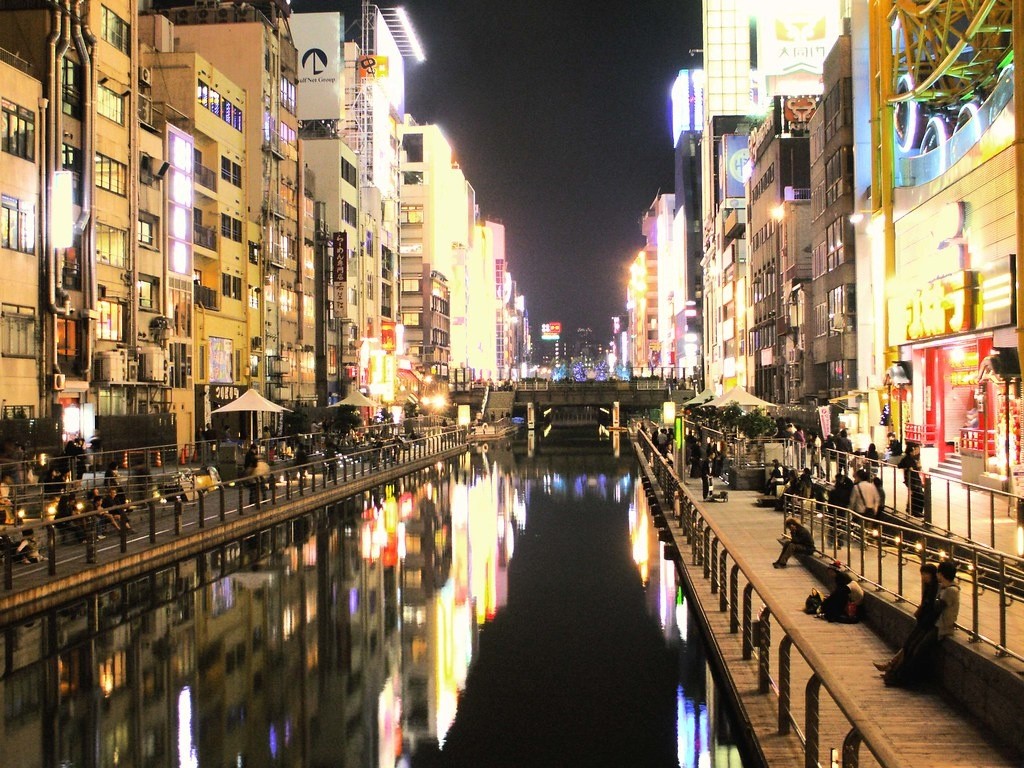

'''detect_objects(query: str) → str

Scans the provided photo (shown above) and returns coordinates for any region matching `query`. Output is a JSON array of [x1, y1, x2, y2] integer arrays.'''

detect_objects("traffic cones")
[[191, 449, 200, 463], [121, 450, 129, 469], [179, 448, 188, 465], [153, 450, 162, 467]]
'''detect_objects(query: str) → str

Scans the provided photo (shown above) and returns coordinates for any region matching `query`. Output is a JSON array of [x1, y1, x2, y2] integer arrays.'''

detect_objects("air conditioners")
[[48, 374, 64, 390], [99, 349, 128, 383], [139, 354, 168, 383], [136, 67, 150, 84]]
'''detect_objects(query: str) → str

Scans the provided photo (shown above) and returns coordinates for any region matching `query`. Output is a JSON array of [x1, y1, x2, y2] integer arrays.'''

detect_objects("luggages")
[[758, 495, 779, 507]]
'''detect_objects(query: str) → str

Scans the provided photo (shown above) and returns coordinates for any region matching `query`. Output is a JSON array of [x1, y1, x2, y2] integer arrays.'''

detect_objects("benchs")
[[61, 516, 112, 546]]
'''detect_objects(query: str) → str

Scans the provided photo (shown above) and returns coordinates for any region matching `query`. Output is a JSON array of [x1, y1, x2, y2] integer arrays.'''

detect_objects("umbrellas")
[[696, 386, 777, 430], [327, 388, 377, 423], [209, 388, 294, 445], [680, 388, 721, 411]]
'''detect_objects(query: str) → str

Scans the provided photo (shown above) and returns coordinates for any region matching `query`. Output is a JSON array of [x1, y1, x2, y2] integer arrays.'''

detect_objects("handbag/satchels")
[[865, 508, 874, 522], [806, 588, 823, 614]]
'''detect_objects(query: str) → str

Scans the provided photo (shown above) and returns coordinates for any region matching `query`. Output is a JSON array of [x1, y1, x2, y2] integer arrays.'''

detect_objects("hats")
[[829, 561, 841, 572]]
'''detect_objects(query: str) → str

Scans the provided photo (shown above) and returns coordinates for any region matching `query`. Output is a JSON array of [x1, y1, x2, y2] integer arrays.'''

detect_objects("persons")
[[638, 418, 735, 507], [873, 557, 960, 689], [815, 561, 864, 623], [2, 411, 513, 570], [770, 519, 815, 571], [488, 373, 698, 398], [752, 417, 926, 549]]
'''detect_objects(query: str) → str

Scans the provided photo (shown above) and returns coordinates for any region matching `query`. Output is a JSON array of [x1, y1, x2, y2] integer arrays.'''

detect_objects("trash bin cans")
[[649, 409, 661, 423]]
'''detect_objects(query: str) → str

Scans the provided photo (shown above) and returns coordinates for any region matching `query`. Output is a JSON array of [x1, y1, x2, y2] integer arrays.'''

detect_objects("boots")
[[873, 648, 904, 671]]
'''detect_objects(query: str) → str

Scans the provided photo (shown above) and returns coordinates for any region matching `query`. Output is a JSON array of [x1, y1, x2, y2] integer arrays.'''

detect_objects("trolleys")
[[707, 476, 728, 502]]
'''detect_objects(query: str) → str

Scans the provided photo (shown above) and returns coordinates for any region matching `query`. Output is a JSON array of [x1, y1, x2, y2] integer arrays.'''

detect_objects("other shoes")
[[127, 528, 138, 533], [98, 536, 106, 539], [119, 530, 128, 536], [773, 560, 786, 568]]
